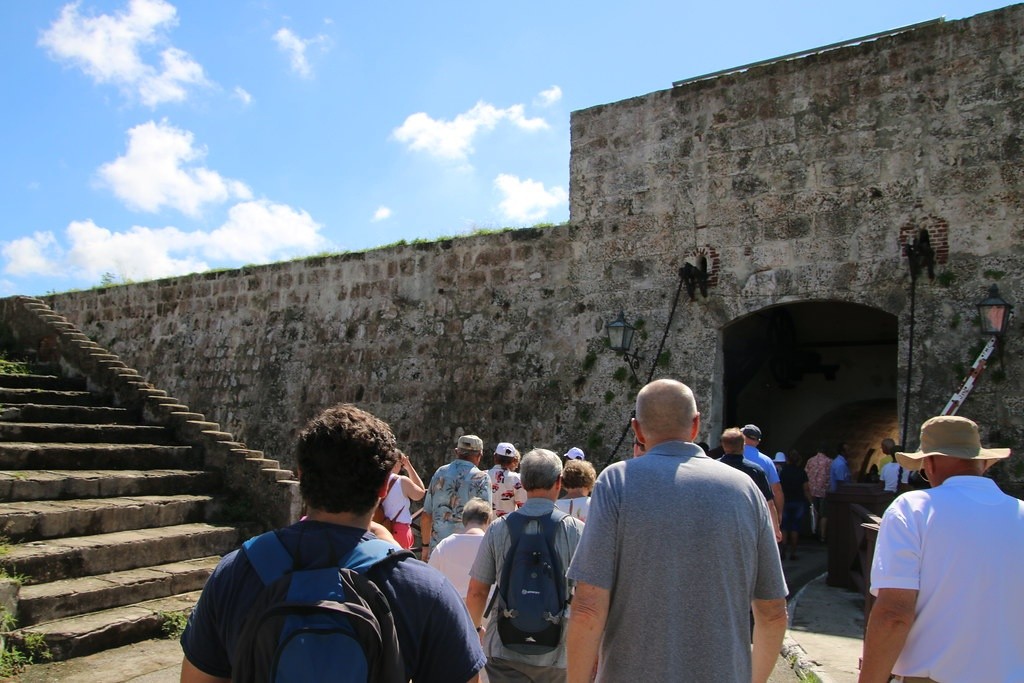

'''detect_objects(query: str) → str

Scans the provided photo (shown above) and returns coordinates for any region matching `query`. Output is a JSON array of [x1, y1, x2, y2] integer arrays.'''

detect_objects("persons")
[[421, 435, 492, 562], [557, 447, 596, 524], [429, 498, 497, 631], [179, 406, 487, 683], [381, 449, 425, 549], [566, 379, 788, 683], [466, 449, 584, 683], [632, 437, 646, 457], [483, 442, 529, 523], [859, 415, 1024, 683], [868, 438, 930, 515], [698, 426, 854, 644]]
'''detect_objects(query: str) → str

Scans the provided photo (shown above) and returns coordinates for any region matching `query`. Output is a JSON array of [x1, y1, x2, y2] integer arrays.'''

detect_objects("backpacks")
[[230, 530, 418, 683], [496, 510, 574, 655], [372, 475, 405, 536]]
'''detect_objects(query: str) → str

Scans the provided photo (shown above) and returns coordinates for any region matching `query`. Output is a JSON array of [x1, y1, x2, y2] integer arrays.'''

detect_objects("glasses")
[[918, 457, 929, 482]]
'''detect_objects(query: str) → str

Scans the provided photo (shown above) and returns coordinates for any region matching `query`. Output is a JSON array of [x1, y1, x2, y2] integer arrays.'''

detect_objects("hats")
[[564, 448, 584, 460], [494, 443, 516, 458], [740, 424, 762, 440], [895, 416, 1011, 470], [772, 452, 786, 462], [457, 435, 483, 451]]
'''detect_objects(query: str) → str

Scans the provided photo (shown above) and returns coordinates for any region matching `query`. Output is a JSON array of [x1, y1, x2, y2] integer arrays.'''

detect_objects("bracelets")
[[422, 543, 429, 547], [476, 625, 482, 632]]
